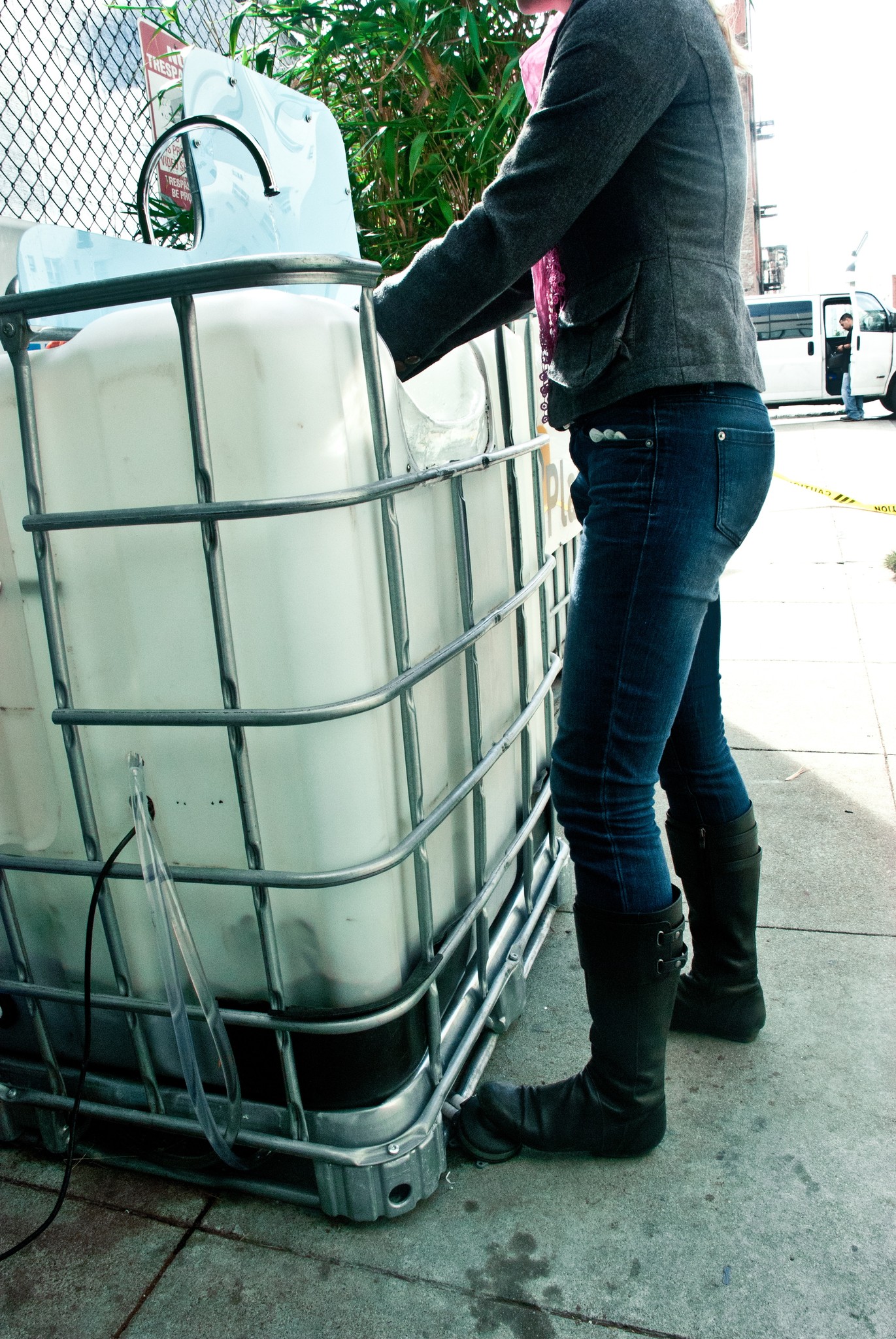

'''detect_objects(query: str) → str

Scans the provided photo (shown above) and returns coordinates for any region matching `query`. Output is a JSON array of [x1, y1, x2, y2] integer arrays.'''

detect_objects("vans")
[[743, 289, 896, 416]]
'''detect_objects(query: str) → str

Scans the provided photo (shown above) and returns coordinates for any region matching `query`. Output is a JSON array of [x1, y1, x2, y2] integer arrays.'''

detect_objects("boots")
[[477, 884, 688, 1153], [667, 798, 765, 1044]]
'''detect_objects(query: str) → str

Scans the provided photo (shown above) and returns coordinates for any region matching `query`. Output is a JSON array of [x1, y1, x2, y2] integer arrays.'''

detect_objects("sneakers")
[[840, 416, 855, 421]]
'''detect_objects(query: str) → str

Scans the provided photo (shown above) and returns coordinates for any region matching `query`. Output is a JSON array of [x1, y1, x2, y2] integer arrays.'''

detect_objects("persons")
[[837, 313, 865, 421], [368, 0, 776, 1167]]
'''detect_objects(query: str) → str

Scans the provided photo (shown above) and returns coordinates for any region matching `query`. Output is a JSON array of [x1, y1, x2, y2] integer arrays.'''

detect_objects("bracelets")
[[843, 345, 846, 350]]
